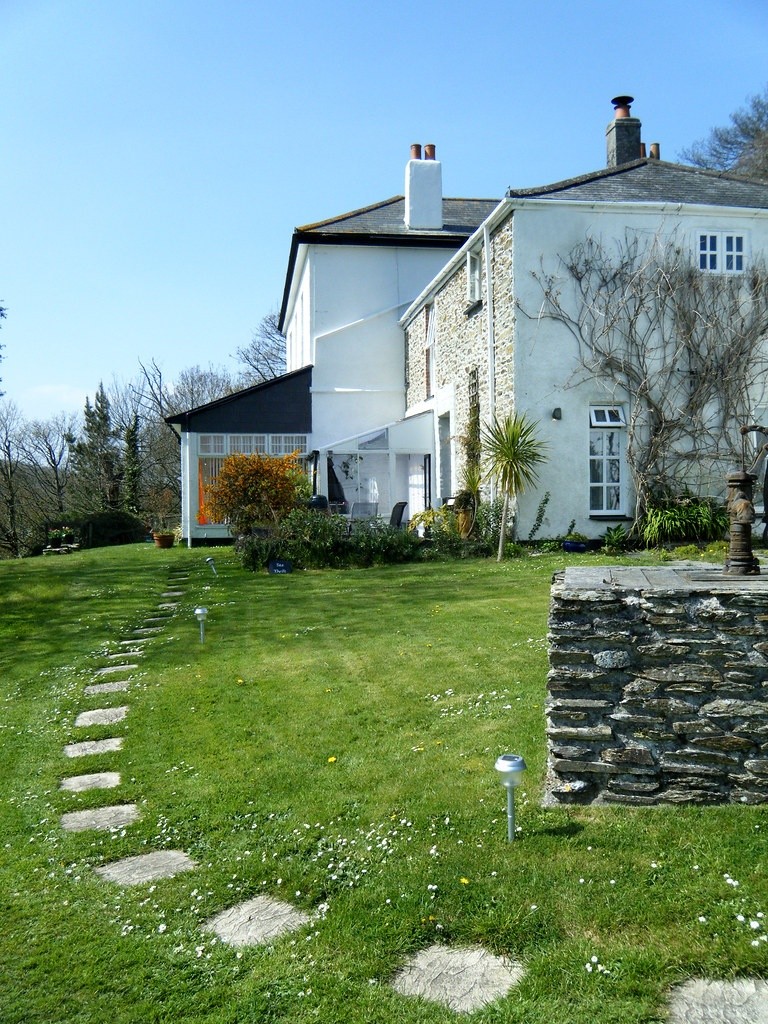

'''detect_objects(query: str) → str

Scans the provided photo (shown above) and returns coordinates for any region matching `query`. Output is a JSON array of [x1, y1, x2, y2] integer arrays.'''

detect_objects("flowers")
[[49, 529, 62, 538], [63, 527, 75, 534]]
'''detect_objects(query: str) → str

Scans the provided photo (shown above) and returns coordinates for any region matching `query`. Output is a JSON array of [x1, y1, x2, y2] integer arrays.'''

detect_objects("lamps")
[[552, 408, 562, 421], [495, 752, 529, 789], [193, 605, 207, 621], [206, 556, 215, 567]]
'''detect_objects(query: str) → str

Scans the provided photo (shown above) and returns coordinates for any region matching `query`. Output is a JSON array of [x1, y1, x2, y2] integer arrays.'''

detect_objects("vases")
[[51, 537, 61, 548], [63, 534, 75, 545]]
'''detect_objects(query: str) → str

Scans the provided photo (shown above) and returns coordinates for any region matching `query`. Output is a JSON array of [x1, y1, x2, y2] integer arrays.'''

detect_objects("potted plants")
[[454, 490, 477, 540], [565, 533, 587, 551], [153, 528, 175, 547], [599, 526, 626, 554]]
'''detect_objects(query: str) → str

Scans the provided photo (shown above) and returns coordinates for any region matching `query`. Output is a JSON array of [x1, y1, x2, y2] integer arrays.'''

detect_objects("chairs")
[[331, 495, 409, 532]]
